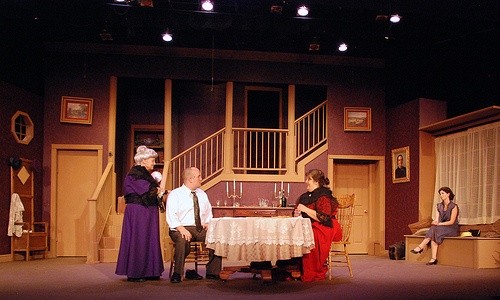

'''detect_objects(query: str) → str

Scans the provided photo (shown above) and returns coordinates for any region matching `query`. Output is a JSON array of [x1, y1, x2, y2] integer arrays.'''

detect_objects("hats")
[[460, 232, 473, 237]]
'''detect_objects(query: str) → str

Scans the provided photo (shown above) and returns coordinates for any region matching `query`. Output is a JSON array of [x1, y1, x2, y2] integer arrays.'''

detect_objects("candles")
[[275, 182, 277, 192], [281, 181, 283, 190], [240, 182, 242, 193], [226, 181, 228, 192], [234, 179, 235, 191], [287, 183, 289, 194]]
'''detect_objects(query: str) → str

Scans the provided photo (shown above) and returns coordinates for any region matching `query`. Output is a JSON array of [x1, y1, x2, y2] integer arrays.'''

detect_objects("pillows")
[[414, 227, 430, 236]]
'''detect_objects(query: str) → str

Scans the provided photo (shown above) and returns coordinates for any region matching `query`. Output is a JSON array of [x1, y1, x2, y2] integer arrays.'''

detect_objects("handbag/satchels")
[[469, 229, 481, 237]]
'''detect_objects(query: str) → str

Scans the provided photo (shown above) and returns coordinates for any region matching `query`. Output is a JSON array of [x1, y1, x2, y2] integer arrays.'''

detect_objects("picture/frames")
[[59, 96, 93, 124], [343, 106, 372, 131], [392, 146, 411, 184]]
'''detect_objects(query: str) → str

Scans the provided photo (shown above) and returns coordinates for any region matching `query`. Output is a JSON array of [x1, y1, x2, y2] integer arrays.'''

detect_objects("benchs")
[[402, 219, 500, 268]]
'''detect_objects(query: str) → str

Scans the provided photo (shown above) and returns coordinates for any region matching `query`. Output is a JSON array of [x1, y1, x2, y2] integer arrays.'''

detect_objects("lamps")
[[112, 0, 402, 53]]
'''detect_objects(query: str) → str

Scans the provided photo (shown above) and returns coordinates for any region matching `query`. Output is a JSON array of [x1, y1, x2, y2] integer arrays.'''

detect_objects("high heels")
[[425, 258, 437, 265], [410, 246, 423, 254]]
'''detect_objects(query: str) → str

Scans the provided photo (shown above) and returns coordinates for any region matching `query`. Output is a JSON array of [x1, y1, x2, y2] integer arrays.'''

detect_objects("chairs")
[[9, 158, 48, 262], [325, 194, 355, 281]]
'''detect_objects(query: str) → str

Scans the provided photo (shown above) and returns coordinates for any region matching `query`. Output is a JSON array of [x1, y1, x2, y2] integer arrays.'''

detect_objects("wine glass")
[[224, 201, 227, 207]]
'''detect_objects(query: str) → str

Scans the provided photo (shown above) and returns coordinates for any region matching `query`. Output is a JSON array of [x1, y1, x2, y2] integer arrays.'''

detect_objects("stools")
[[169, 241, 204, 279]]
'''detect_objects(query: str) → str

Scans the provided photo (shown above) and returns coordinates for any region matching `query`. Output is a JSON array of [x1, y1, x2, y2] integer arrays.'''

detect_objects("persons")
[[115, 146, 165, 282], [165, 167, 222, 283], [410, 187, 459, 265], [292, 169, 342, 282]]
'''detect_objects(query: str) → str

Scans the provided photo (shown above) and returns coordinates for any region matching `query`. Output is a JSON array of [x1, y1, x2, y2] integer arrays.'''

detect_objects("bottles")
[[260, 199, 278, 208], [280, 197, 282, 207], [282, 190, 286, 207]]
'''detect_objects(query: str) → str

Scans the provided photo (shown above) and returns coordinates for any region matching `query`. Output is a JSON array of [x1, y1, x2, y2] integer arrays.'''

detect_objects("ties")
[[192, 192, 202, 234]]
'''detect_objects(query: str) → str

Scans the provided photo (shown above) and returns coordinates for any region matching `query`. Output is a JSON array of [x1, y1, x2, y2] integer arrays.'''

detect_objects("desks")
[[208, 217, 316, 283]]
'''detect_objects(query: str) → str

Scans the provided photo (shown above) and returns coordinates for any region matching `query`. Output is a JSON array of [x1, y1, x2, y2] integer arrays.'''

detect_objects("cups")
[[217, 200, 220, 207]]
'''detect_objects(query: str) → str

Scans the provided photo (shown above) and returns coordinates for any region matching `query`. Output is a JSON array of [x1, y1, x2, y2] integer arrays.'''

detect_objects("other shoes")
[[127, 277, 144, 282], [185, 270, 203, 280], [170, 272, 181, 283], [147, 276, 160, 280]]
